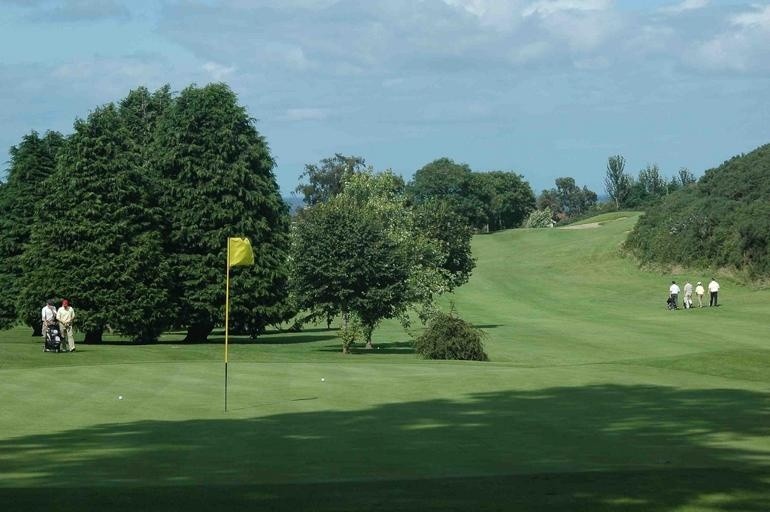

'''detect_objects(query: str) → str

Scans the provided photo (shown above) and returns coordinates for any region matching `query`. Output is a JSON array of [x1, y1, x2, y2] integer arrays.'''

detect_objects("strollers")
[[43, 319, 63, 353]]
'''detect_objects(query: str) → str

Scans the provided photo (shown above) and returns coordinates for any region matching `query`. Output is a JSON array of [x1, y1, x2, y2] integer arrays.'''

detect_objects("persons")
[[41, 299, 56, 336], [56, 300, 77, 352], [669, 280, 680, 310], [695, 282, 704, 307], [708, 278, 720, 306], [683, 280, 693, 309], [51, 329, 60, 342]]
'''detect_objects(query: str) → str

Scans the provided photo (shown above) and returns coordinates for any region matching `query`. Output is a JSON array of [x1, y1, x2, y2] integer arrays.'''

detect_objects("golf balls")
[[322, 379, 325, 382], [119, 395, 123, 399]]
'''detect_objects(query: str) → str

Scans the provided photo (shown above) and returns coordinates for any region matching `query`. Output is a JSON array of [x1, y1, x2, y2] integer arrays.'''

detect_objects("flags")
[[229, 237, 255, 267]]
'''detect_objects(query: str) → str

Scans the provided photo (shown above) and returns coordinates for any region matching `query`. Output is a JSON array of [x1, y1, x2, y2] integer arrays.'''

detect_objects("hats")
[[63, 300, 68, 307], [47, 299, 53, 305]]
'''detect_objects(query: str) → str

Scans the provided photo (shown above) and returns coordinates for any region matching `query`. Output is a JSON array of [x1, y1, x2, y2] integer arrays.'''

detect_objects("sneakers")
[[671, 305, 718, 310], [62, 346, 75, 352]]
